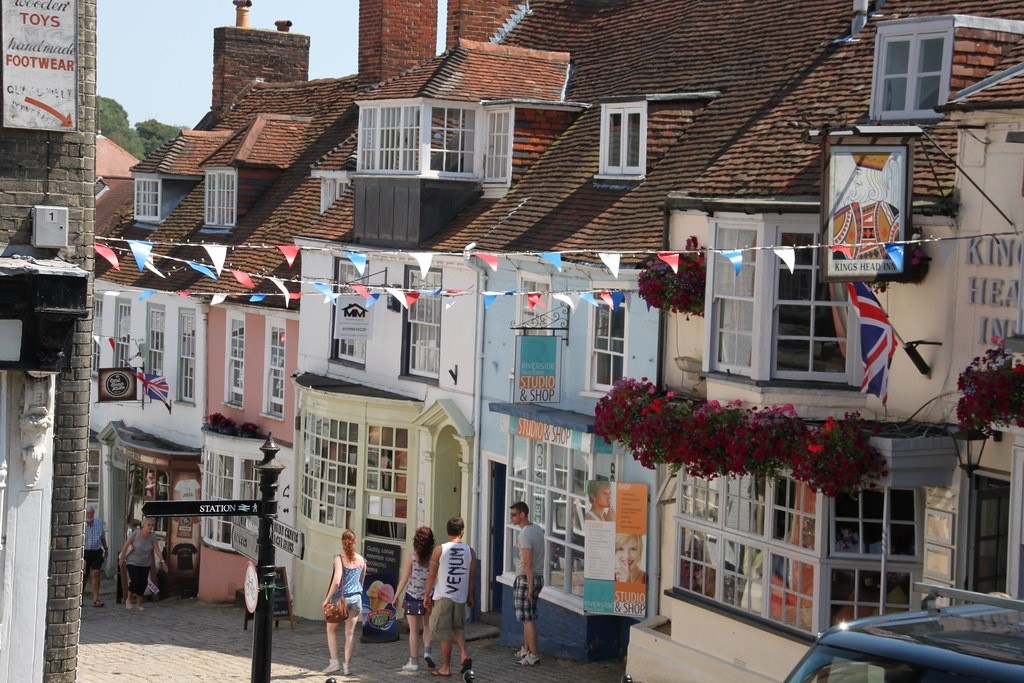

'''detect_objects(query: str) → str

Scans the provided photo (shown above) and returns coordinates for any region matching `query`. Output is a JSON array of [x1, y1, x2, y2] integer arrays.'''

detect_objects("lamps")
[[673, 355, 701, 381]]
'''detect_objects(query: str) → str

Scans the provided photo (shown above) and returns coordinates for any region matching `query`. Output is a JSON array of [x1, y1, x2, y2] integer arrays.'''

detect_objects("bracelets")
[[105, 548, 107, 550], [161, 560, 165, 563]]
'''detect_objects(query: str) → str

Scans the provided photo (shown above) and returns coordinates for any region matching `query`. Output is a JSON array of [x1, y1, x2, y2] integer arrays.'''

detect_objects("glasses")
[[511, 512, 522, 516]]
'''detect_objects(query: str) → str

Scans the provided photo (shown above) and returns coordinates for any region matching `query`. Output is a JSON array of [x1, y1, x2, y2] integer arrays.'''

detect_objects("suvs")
[[785, 580, 1024, 683]]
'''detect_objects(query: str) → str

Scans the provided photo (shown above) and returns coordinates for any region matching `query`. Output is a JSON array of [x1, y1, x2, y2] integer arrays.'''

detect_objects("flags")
[[846, 281, 898, 407], [124, 359, 169, 402]]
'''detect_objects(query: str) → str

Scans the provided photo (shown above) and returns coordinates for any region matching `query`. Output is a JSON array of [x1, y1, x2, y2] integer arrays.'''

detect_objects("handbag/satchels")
[[405, 600, 425, 616], [323, 594, 349, 623]]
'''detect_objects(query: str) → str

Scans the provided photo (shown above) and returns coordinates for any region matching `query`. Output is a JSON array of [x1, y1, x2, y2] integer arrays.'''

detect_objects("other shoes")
[[136, 606, 146, 613], [125, 600, 133, 612], [93, 600, 105, 607]]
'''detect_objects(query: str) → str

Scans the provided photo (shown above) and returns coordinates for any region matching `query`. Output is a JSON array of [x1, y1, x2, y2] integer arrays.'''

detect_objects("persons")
[[510, 502, 545, 666], [585, 481, 616, 522], [615, 533, 646, 584], [322, 530, 366, 675], [423, 517, 477, 676], [81, 506, 108, 607], [118, 519, 168, 611], [392, 526, 436, 671]]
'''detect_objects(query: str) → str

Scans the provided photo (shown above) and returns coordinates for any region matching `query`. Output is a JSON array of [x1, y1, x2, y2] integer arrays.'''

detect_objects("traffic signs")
[[142, 500, 260, 516]]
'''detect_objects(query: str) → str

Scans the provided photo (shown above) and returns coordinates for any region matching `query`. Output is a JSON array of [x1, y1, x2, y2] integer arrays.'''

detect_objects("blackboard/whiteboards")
[[245, 567, 293, 620]]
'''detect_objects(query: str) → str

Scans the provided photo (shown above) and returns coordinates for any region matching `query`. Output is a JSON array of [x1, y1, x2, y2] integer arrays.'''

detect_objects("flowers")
[[209, 412, 256, 433], [596, 245, 1023, 503]]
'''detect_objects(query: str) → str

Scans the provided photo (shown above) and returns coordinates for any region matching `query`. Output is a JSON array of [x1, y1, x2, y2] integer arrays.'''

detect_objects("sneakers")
[[423, 652, 437, 668], [514, 651, 541, 666], [322, 659, 341, 673], [343, 661, 353, 675], [402, 657, 420, 671], [514, 649, 529, 659]]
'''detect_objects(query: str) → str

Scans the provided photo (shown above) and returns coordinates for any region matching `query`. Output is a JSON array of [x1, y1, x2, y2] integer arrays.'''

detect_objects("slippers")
[[430, 667, 451, 677], [460, 657, 473, 674]]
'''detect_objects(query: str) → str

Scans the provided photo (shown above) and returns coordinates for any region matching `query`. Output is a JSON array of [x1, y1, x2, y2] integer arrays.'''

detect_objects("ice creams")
[[366, 580, 394, 610]]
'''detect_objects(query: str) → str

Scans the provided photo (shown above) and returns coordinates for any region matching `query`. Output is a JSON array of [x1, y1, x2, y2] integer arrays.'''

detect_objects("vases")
[[218, 426, 238, 435], [209, 424, 219, 431], [239, 431, 256, 437]]
